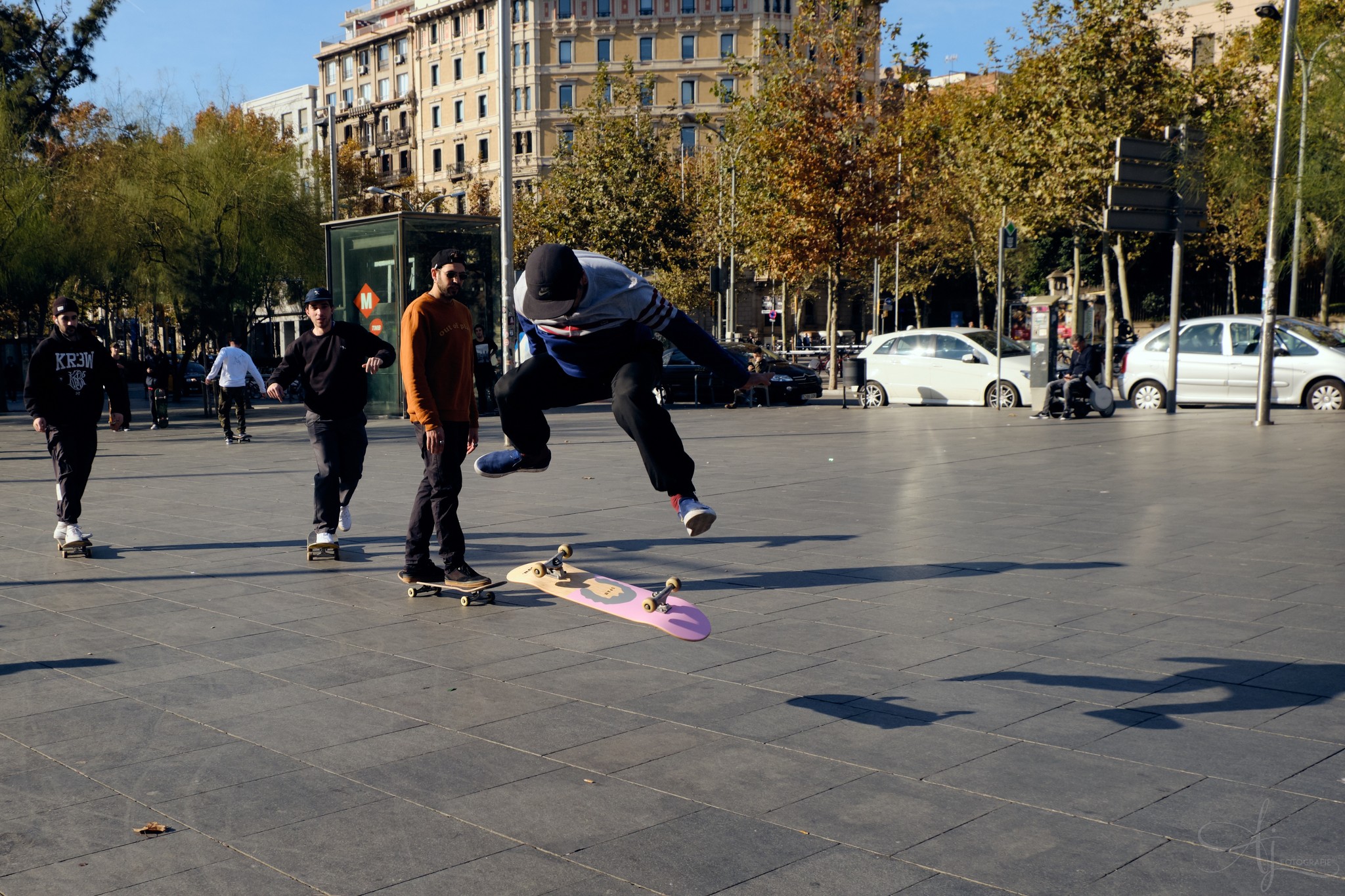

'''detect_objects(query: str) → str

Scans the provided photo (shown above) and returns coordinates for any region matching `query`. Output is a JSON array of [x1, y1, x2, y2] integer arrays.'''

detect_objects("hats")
[[425, 249, 471, 286], [53, 296, 79, 316], [523, 244, 581, 318], [303, 287, 332, 302], [152, 340, 160, 345]]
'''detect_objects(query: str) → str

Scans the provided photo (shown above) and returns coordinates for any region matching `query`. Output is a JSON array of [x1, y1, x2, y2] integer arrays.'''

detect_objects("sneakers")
[[53, 524, 92, 540], [316, 532, 334, 544], [1061, 413, 1072, 421], [1029, 411, 1049, 419], [65, 524, 84, 544], [338, 506, 351, 531]]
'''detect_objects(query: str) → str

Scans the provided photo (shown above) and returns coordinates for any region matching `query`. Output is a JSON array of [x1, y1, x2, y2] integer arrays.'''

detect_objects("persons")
[[1030, 334, 1098, 420], [400, 248, 492, 588], [969, 322, 974, 328], [24, 296, 124, 543], [984, 322, 991, 330], [266, 287, 396, 544], [144, 339, 171, 429], [473, 243, 775, 537], [474, 324, 497, 415], [803, 333, 811, 357], [103, 342, 132, 431], [866, 330, 873, 344], [798, 335, 803, 357], [725, 346, 768, 409], [1015, 309, 1026, 328], [747, 332, 762, 346], [206, 334, 267, 444], [1112, 318, 1132, 370]]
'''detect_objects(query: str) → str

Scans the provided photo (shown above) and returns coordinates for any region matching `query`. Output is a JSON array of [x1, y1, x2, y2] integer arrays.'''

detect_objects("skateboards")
[[57, 539, 92, 558], [155, 388, 169, 429], [506, 543, 711, 642], [224, 433, 252, 443], [397, 568, 508, 606], [307, 530, 340, 561]]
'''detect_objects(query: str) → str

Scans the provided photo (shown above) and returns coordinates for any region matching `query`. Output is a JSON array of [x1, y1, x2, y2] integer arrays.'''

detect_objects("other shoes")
[[225, 438, 233, 444], [677, 497, 716, 537], [444, 562, 492, 587], [239, 432, 246, 437], [123, 426, 130, 431], [151, 424, 159, 430], [402, 559, 445, 583], [474, 448, 552, 477]]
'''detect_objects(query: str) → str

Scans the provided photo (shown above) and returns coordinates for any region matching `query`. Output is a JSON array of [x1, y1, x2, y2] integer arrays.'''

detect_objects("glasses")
[[437, 268, 469, 281]]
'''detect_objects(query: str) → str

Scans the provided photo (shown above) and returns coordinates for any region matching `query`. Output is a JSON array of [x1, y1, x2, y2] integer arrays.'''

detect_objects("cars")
[[855, 327, 1076, 406], [1119, 316, 1345, 408], [164, 350, 217, 397], [658, 343, 824, 405]]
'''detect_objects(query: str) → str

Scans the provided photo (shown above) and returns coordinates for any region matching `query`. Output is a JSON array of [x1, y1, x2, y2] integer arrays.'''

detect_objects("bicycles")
[[759, 329, 861, 378], [245, 376, 263, 399]]
